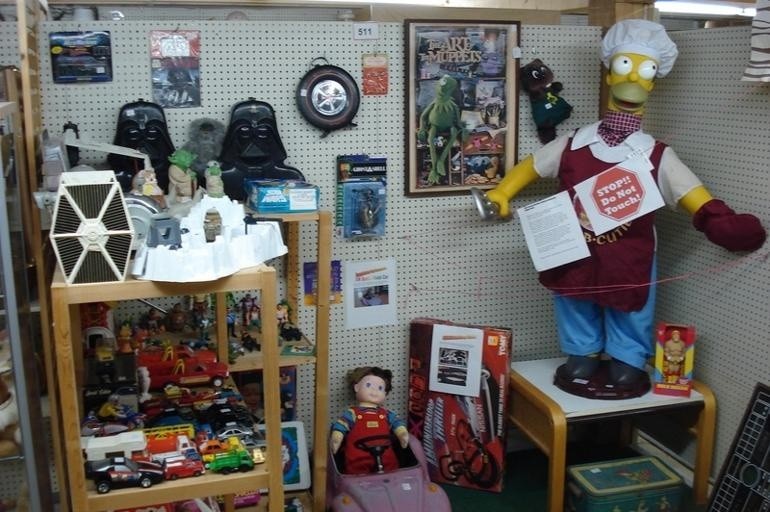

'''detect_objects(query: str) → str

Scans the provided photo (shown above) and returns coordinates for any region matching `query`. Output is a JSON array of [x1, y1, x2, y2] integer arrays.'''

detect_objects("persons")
[[222, 100, 307, 203], [331, 365, 410, 474], [486, 19, 766, 393], [144, 294, 289, 338], [108, 103, 176, 196]]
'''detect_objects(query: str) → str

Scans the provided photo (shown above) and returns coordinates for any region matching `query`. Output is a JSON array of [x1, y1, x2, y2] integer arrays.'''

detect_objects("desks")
[[507, 353, 717, 511]]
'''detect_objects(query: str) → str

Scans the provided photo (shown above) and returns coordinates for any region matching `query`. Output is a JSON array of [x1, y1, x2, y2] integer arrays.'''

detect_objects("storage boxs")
[[566, 455, 683, 512]]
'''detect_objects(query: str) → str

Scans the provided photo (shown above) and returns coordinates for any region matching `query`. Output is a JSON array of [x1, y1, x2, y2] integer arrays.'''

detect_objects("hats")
[[598, 17, 680, 80]]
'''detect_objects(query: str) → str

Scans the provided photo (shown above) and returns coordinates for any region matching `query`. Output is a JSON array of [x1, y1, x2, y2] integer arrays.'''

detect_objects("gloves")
[[689, 199, 765, 253]]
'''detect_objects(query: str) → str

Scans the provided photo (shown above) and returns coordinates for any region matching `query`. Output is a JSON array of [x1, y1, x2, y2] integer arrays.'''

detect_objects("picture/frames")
[[403, 20, 521, 200]]
